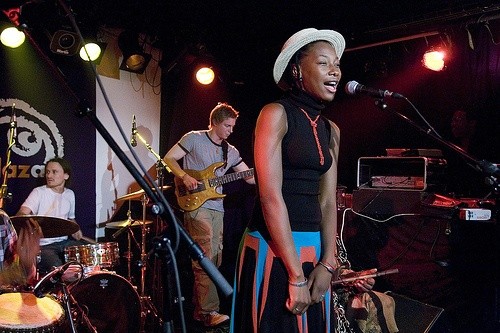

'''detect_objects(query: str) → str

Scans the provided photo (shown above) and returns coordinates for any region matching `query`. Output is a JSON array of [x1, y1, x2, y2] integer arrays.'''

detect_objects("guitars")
[[173, 162, 255, 213]]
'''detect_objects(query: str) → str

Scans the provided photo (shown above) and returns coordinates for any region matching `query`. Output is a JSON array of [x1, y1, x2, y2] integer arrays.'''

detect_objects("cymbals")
[[8, 214, 80, 238], [112, 186, 171, 203], [104, 219, 155, 230]]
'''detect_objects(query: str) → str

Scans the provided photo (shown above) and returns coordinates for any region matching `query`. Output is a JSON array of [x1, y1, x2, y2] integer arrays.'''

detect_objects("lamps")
[[49, 26, 107, 67], [182, 50, 218, 87], [120, 44, 153, 75]]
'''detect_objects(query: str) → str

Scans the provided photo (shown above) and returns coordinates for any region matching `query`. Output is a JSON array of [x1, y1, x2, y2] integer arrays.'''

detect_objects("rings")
[[29, 232, 34, 235], [295, 307, 300, 313]]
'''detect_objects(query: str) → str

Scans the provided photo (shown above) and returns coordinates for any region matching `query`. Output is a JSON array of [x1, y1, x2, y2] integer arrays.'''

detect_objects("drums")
[[33, 265, 143, 333], [0, 286, 67, 333], [65, 241, 120, 268]]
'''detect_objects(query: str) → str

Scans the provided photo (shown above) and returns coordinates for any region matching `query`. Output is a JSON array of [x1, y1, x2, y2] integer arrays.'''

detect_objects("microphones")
[[112, 220, 135, 238], [50, 262, 70, 283], [345, 81, 404, 98], [131, 115, 137, 147]]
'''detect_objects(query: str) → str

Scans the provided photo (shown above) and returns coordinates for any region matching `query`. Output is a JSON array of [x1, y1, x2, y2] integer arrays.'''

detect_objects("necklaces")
[[300, 107, 325, 165]]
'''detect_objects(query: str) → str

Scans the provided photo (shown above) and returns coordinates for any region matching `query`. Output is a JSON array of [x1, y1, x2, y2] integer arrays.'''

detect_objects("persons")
[[434, 111, 472, 176], [230, 27, 345, 333], [0, 157, 82, 295], [163, 103, 257, 325]]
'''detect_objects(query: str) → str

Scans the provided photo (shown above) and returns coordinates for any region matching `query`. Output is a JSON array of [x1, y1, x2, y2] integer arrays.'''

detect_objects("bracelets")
[[288, 277, 308, 287], [178, 171, 187, 179], [320, 262, 334, 275]]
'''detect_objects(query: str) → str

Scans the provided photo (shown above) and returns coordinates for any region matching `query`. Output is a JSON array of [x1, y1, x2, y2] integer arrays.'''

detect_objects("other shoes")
[[203, 311, 229, 325]]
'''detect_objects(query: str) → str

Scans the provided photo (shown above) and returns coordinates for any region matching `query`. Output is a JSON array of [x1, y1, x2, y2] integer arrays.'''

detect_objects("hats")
[[273, 28, 345, 90]]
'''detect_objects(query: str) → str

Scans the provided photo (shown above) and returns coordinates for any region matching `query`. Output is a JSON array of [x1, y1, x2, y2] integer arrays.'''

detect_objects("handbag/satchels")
[[355, 290, 399, 333]]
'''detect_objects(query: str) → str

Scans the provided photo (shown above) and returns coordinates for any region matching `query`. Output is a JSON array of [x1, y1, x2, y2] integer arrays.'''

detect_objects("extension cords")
[[460, 209, 491, 220]]
